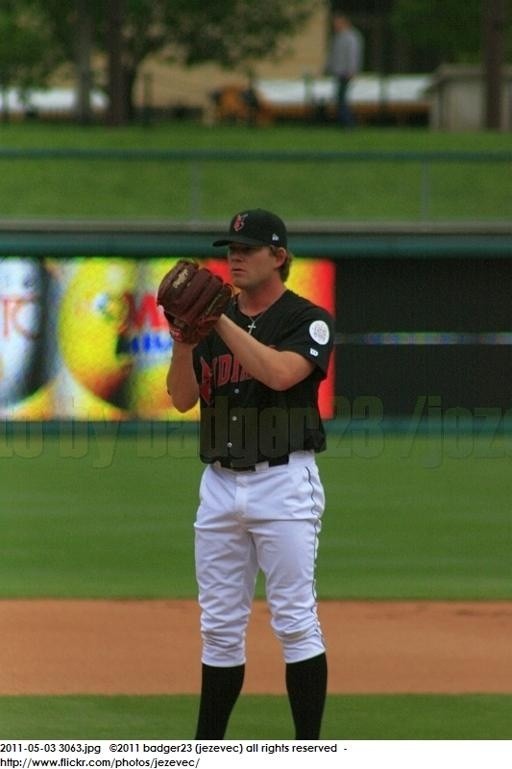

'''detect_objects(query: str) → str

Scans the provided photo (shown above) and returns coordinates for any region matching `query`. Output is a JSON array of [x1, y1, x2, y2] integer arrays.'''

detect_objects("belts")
[[218, 453, 290, 473]]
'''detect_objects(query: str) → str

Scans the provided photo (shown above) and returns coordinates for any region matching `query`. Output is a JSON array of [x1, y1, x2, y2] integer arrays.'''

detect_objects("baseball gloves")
[[154, 259, 233, 342]]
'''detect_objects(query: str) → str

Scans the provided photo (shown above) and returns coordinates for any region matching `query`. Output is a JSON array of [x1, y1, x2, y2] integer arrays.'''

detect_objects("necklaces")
[[232, 290, 281, 338]]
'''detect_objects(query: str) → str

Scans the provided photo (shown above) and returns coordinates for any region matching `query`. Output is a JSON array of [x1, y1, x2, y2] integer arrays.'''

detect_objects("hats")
[[212, 208, 288, 249]]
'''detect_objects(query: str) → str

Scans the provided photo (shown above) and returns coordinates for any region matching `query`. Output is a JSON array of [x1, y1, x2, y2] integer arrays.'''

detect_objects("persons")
[[155, 209, 338, 739], [234, 64, 262, 127], [323, 11, 368, 129]]
